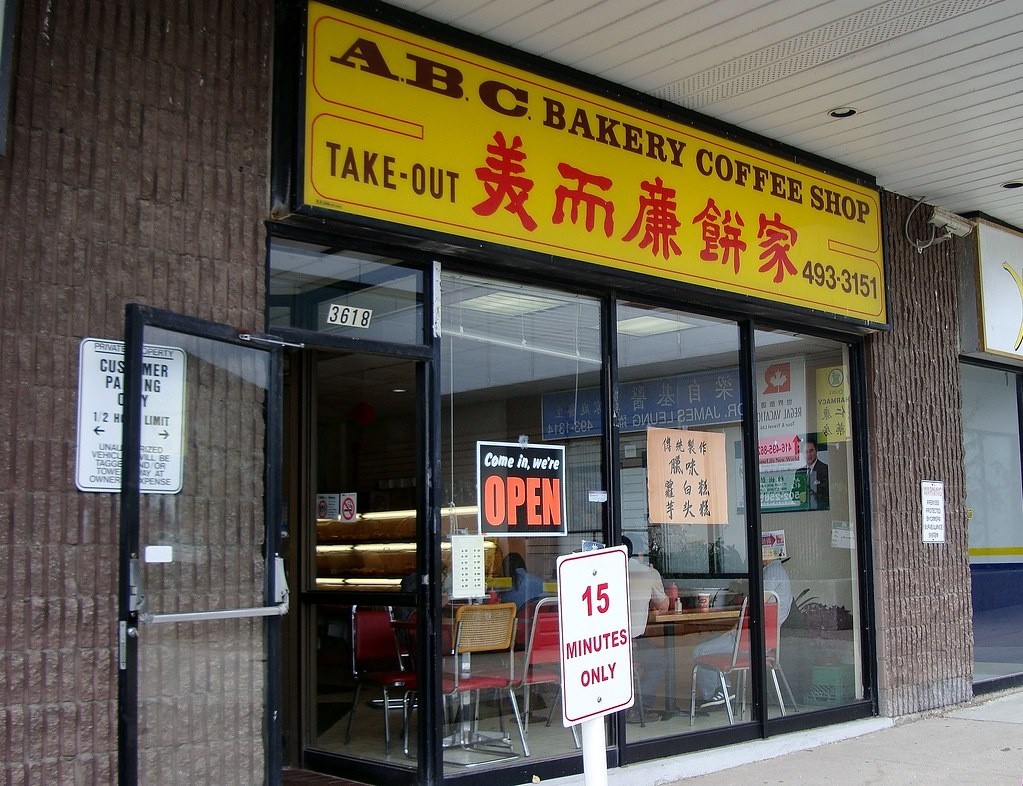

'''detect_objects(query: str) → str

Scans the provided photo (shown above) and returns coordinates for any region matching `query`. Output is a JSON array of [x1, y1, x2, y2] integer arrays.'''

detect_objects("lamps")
[[441, 283, 601, 365]]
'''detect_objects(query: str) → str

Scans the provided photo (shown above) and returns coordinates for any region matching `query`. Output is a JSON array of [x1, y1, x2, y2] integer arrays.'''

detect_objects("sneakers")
[[694, 685, 735, 712]]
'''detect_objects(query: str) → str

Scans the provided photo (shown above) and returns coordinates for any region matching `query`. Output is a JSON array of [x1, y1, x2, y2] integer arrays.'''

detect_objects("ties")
[[807, 467, 811, 474]]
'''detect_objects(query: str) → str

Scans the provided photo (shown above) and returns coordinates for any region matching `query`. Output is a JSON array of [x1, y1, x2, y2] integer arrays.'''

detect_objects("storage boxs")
[[802, 663, 855, 707]]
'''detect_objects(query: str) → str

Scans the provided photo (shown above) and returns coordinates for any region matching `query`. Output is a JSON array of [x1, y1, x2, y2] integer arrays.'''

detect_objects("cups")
[[698, 593, 711, 613], [487, 590, 497, 604]]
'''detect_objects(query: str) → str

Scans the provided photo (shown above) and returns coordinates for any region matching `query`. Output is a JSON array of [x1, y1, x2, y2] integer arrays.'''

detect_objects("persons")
[[693, 548, 792, 708], [791, 438, 828, 508], [496, 552, 544, 609], [395, 560, 450, 648], [624, 533, 669, 725]]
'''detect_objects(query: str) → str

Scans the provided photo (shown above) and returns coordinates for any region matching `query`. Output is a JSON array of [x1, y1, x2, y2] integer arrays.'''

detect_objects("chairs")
[[343, 605, 466, 755], [403, 601, 530, 768], [469, 596, 582, 757], [689, 591, 788, 727]]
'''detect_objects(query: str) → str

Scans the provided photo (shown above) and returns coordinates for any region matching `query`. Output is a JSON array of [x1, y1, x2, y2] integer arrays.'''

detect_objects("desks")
[[647, 608, 741, 722]]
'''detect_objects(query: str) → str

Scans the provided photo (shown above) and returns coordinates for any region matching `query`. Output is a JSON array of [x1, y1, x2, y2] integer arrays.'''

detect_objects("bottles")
[[675, 597, 683, 615], [669, 582, 679, 611]]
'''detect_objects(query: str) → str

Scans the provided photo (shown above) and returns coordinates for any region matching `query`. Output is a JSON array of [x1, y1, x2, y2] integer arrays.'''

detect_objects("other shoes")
[[625, 709, 659, 724]]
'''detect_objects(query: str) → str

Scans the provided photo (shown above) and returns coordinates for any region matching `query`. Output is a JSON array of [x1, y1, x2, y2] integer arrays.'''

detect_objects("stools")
[[633, 661, 646, 728], [734, 652, 800, 717]]
